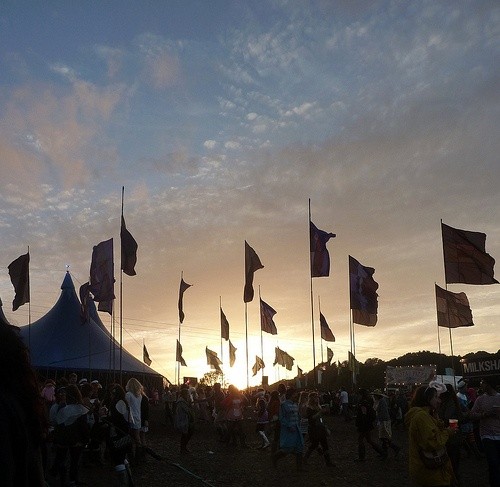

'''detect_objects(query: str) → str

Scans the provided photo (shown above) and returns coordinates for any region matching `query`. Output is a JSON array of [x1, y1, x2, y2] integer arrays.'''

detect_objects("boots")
[[389, 444, 401, 457], [256, 431, 271, 449]]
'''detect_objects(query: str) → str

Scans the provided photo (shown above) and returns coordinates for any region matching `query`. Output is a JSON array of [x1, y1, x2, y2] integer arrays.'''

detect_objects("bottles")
[[102, 404, 108, 414]]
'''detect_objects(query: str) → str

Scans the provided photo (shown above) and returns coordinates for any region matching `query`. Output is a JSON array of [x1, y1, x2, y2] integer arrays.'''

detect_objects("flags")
[[252, 356, 265, 377], [349, 255, 378, 326], [89, 217, 138, 316], [144, 345, 151, 366], [176, 341, 187, 366], [261, 300, 278, 334], [278, 349, 295, 370], [320, 312, 335, 342], [327, 348, 333, 360], [244, 241, 265, 303], [441, 222, 500, 285], [229, 340, 237, 366], [178, 279, 192, 323], [309, 222, 336, 279], [206, 348, 217, 365], [9, 253, 30, 311], [434, 284, 474, 328], [221, 309, 229, 340]]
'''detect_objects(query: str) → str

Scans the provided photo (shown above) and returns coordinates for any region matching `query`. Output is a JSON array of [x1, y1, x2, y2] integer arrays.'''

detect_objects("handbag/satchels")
[[418, 444, 449, 468]]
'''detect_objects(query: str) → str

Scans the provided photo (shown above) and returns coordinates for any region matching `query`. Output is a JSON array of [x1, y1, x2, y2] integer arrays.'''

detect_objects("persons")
[[0, 319, 500, 487]]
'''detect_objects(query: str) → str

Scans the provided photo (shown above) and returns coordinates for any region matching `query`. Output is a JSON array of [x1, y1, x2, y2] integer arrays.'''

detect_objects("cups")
[[449, 418, 458, 434]]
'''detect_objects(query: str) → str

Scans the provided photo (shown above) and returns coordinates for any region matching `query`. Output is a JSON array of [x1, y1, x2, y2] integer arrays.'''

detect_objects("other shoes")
[[240, 443, 250, 448], [354, 459, 365, 462], [383, 453, 387, 460], [272, 456, 278, 469], [326, 463, 336, 467], [180, 447, 190, 455], [48, 456, 147, 487], [295, 467, 309, 472]]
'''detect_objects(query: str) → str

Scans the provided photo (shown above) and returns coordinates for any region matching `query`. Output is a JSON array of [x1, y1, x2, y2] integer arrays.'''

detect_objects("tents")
[[26, 270, 163, 398]]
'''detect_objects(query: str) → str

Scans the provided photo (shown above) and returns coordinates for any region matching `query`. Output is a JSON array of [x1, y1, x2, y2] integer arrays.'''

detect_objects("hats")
[[91, 380, 99, 384], [79, 379, 87, 384]]
